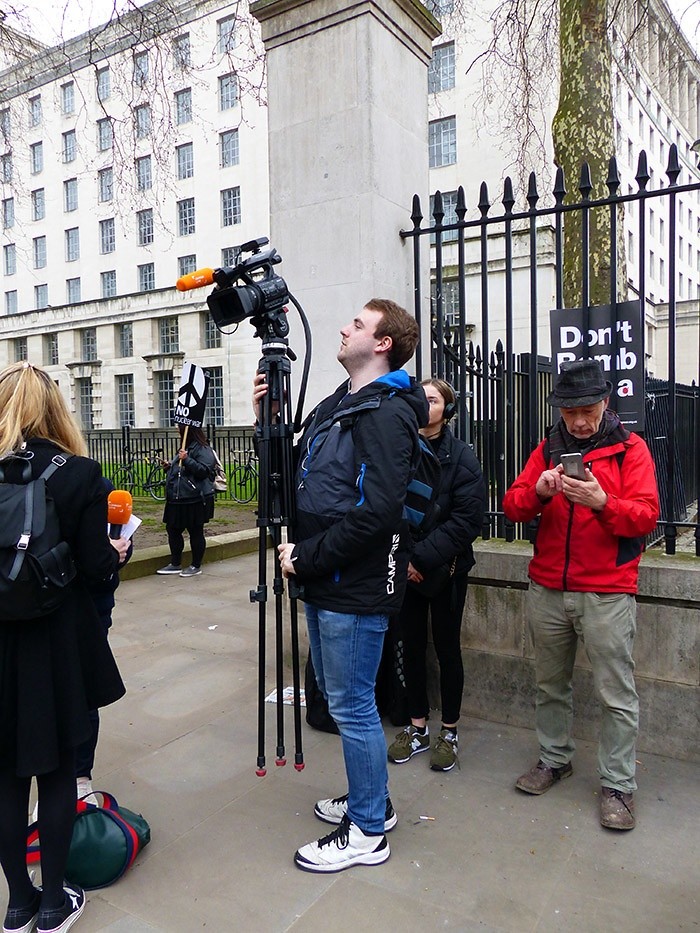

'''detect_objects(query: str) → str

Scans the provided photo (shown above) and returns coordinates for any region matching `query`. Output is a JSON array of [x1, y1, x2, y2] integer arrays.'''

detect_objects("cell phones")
[[561, 452, 586, 482], [156, 457, 167, 465]]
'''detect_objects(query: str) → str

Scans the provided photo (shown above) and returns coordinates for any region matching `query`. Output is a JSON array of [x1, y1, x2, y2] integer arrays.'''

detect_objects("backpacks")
[[192, 445, 228, 492], [0, 452, 76, 611]]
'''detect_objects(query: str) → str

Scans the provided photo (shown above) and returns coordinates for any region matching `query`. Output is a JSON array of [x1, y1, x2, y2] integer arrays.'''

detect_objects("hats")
[[545, 360, 613, 408]]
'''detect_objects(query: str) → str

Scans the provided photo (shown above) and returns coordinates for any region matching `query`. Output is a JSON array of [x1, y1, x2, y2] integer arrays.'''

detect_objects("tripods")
[[246, 305, 306, 778]]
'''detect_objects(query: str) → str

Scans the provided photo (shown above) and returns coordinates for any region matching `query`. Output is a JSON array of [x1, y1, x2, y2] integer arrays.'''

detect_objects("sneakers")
[[294, 812, 390, 873], [515, 754, 572, 795], [2, 885, 44, 933], [387, 726, 430, 764], [430, 731, 461, 772], [314, 790, 398, 833], [36, 883, 86, 933], [601, 783, 635, 829]]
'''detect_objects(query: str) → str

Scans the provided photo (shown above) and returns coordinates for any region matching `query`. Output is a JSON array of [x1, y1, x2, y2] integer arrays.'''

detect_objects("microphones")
[[107, 489, 133, 540], [176, 267, 219, 293]]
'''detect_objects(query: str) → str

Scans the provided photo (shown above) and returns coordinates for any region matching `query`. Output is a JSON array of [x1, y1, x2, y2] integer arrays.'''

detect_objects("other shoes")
[[180, 568, 202, 576], [157, 563, 183, 574]]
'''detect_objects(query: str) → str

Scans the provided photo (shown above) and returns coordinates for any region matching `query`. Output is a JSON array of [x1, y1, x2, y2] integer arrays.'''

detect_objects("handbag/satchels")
[[23, 790, 150, 892]]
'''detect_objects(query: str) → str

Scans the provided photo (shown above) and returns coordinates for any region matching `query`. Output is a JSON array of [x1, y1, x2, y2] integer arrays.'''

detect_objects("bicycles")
[[109, 445, 167, 501], [227, 448, 260, 504]]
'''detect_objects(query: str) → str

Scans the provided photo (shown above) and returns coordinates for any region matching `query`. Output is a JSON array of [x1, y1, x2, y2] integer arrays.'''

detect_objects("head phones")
[[443, 379, 458, 420]]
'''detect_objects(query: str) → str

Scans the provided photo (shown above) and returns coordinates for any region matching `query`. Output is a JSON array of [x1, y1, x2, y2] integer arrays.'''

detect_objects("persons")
[[0, 358, 133, 933], [253, 297, 430, 874], [500, 359, 660, 831], [156, 422, 218, 578], [386, 377, 486, 771]]
